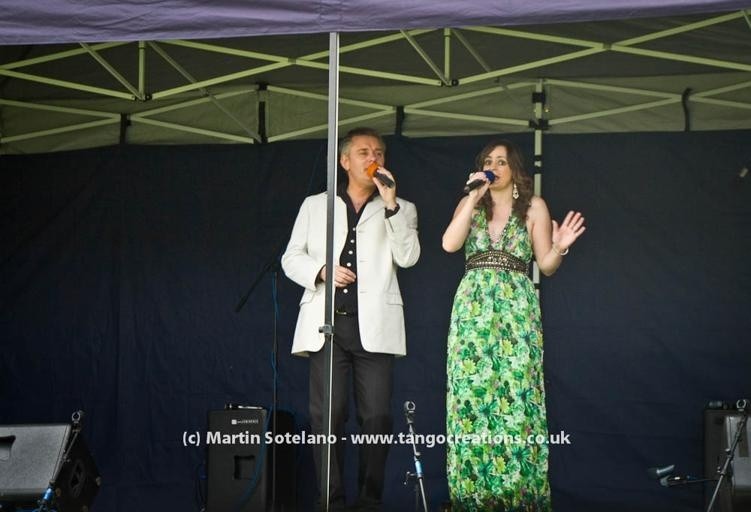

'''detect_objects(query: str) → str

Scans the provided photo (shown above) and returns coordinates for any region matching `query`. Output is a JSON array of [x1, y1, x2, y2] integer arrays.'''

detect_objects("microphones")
[[646, 464, 676, 479], [465, 170, 495, 193], [366, 162, 395, 188], [402, 399, 417, 411]]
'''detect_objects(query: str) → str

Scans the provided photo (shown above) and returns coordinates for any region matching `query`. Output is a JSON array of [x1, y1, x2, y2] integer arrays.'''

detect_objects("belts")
[[335, 307, 349, 316]]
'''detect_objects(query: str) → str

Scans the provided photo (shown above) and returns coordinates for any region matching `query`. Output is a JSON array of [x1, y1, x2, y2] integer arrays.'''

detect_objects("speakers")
[[206, 407, 297, 512], [0, 421, 105, 512], [701, 409, 751, 512]]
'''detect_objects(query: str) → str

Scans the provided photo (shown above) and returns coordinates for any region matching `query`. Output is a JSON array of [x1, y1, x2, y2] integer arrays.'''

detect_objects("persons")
[[280, 127, 421, 512], [442, 138, 586, 511]]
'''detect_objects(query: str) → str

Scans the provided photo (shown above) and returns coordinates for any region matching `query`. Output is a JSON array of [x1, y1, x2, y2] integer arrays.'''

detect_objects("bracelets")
[[551, 242, 569, 256]]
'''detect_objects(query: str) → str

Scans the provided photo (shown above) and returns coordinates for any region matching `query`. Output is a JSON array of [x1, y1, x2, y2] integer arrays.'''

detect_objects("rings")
[[469, 172, 475, 179]]
